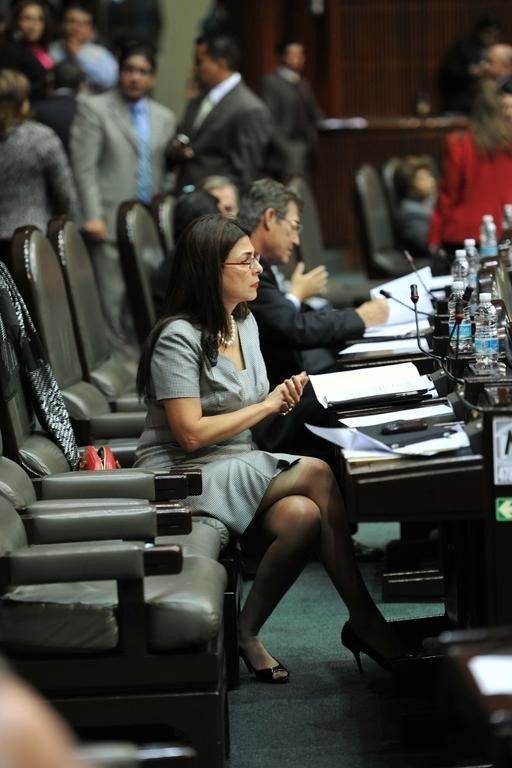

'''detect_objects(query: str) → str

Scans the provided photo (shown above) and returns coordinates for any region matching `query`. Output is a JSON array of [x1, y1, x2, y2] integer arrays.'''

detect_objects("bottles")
[[479, 214, 499, 258], [472, 291, 500, 364], [446, 281, 473, 352], [451, 237, 480, 290]]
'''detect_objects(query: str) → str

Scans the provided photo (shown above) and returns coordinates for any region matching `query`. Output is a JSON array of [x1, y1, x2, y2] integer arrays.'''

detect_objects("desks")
[[334, 231, 512, 768]]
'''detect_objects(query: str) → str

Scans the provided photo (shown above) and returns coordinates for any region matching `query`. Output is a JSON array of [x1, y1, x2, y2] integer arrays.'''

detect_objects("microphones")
[[410, 284, 448, 362], [440, 286, 474, 385], [379, 289, 434, 318], [454, 301, 512, 414], [404, 251, 437, 309]]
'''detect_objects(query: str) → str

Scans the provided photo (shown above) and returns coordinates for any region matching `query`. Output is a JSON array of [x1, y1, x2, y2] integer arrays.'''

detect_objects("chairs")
[[0, 162, 435, 768]]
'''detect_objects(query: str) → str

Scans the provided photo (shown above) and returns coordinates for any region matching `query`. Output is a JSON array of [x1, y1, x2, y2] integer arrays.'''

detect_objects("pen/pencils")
[[394, 389, 427, 397]]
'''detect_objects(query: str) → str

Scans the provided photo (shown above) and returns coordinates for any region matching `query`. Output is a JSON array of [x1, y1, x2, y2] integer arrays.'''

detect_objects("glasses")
[[222, 253, 261, 268], [277, 212, 303, 234]]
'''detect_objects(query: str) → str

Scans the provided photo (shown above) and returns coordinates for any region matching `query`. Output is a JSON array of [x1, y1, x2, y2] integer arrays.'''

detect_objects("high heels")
[[342, 622, 440, 674], [237, 641, 290, 683]]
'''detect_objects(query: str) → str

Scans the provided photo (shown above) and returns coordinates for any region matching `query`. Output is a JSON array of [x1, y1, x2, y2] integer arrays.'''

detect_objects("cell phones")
[[384, 420, 428, 435]]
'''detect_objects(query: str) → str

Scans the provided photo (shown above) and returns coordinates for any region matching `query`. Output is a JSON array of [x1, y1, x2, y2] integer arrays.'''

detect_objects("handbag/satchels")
[[79, 445, 120, 470]]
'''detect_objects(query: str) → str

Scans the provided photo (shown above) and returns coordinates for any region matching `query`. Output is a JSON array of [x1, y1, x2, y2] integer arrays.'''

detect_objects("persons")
[[0, 665, 97, 768], [0, 2, 328, 259], [134, 168, 423, 681], [388, 19, 512, 271]]
